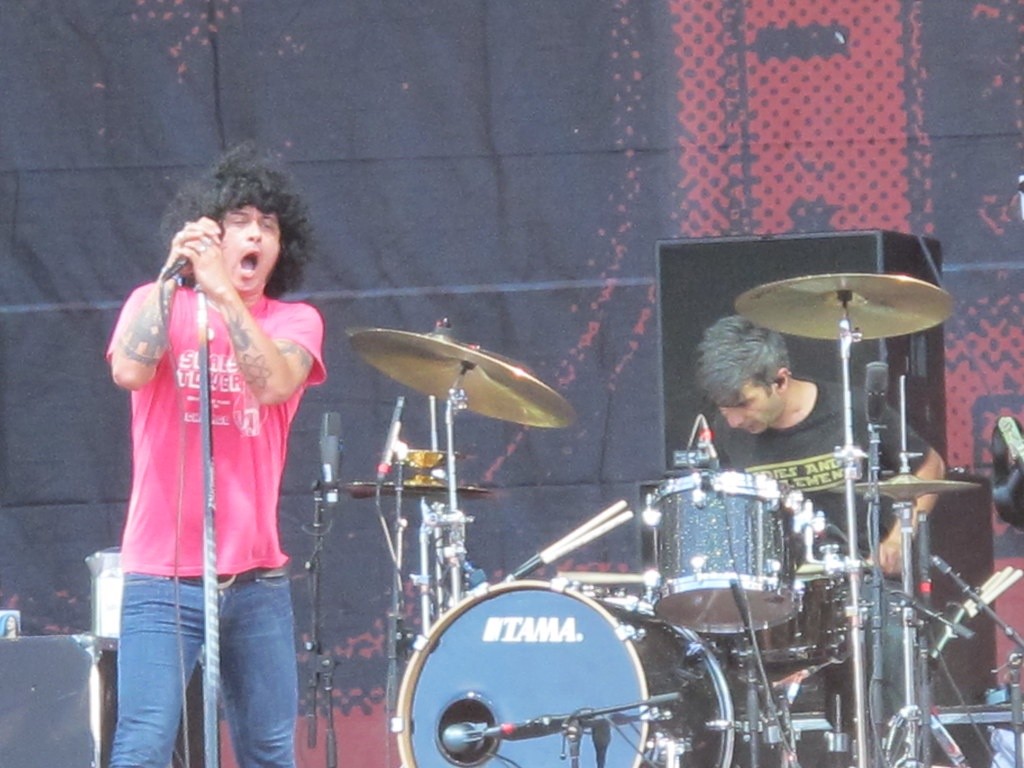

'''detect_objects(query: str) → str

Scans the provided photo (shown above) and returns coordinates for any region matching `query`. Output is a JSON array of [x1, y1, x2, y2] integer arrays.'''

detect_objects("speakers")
[[0, 635, 205, 768], [653, 231, 948, 480], [634, 484, 1000, 768]]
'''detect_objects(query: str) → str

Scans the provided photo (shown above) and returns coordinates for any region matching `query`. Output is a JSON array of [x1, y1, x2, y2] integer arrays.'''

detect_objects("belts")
[[156, 567, 291, 592]]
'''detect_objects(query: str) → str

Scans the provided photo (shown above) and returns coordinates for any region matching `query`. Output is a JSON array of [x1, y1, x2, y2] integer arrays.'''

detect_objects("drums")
[[391, 576, 735, 768], [645, 470, 803, 635], [756, 561, 848, 657]]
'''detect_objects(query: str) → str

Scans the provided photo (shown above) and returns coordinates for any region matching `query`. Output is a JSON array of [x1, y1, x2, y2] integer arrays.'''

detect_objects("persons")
[[104, 139, 331, 767], [691, 314, 949, 584]]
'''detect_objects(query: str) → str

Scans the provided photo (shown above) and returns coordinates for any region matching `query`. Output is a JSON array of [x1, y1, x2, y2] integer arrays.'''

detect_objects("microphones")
[[375, 397, 406, 486], [163, 219, 226, 282], [865, 361, 888, 424], [321, 411, 343, 502], [699, 414, 719, 469]]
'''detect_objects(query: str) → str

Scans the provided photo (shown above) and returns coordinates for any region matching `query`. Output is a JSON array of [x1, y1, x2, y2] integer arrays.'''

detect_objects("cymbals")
[[734, 273, 954, 342], [380, 448, 463, 469], [346, 328, 579, 429], [346, 474, 494, 498], [828, 472, 982, 502]]
